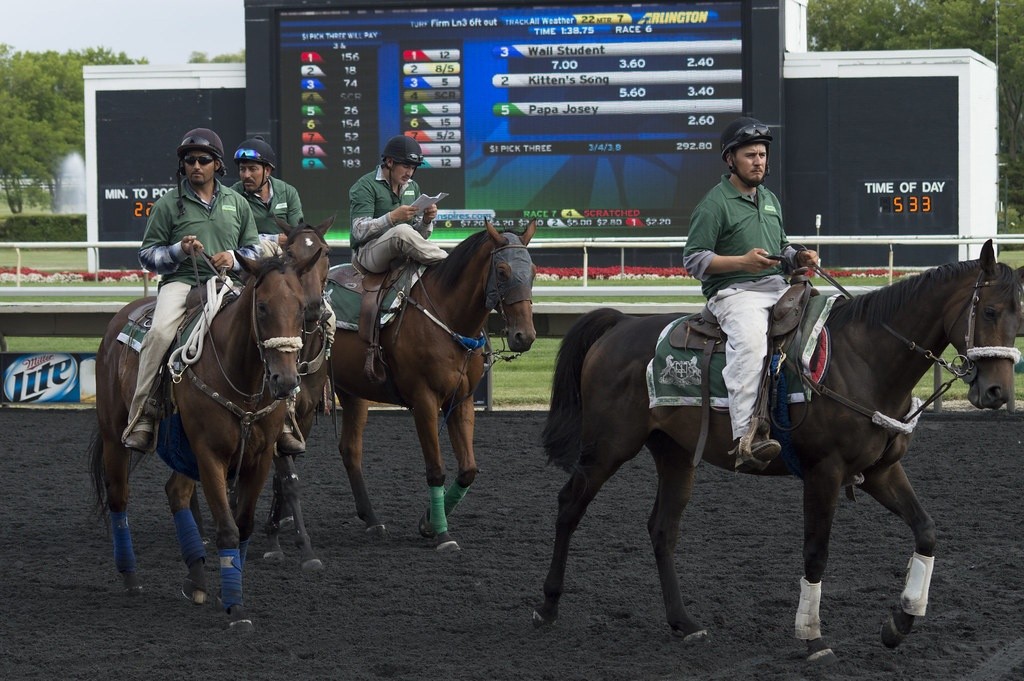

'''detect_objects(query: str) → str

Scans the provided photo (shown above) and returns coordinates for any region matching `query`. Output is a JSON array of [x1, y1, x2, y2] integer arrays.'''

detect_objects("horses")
[[531, 239, 1024, 666], [278, 217, 538, 554], [83, 211, 338, 632]]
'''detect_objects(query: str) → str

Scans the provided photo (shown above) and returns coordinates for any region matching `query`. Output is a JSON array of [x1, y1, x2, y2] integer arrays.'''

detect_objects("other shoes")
[[125, 430, 153, 453], [728, 434, 781, 472], [325, 340, 332, 349], [277, 434, 306, 456]]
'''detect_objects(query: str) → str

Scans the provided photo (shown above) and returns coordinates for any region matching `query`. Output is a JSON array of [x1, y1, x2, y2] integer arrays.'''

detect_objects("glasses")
[[182, 156, 214, 165], [233, 148, 275, 169], [388, 151, 424, 165], [721, 123, 771, 152], [180, 136, 223, 155]]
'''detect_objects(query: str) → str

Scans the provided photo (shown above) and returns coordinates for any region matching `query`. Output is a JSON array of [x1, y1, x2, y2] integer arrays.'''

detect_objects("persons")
[[123, 128, 303, 455], [349, 134, 450, 273], [682, 117, 821, 472]]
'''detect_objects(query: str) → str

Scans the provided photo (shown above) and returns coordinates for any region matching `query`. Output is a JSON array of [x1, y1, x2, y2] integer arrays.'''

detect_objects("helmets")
[[381, 136, 424, 166], [177, 127, 224, 159], [233, 139, 276, 168], [719, 117, 773, 161]]
